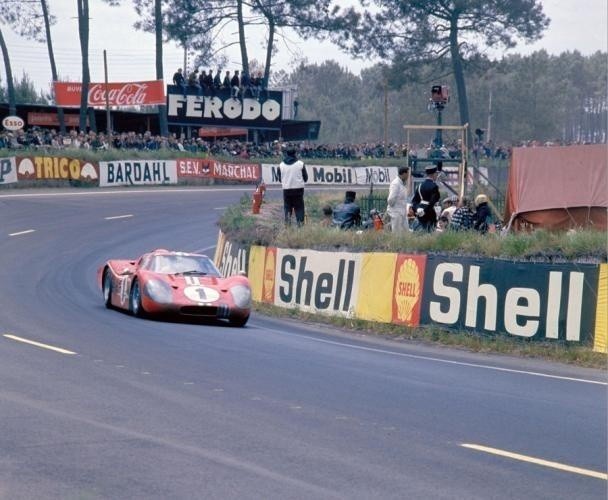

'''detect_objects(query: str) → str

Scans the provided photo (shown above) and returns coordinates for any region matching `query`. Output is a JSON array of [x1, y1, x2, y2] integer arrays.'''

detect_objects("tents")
[[504, 142, 608, 234]]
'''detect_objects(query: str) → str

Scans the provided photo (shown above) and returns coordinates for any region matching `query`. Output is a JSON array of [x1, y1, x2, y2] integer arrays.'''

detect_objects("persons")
[[387, 167, 411, 234], [172, 67, 271, 102], [275, 147, 309, 227], [323, 188, 505, 239], [411, 164, 439, 234]]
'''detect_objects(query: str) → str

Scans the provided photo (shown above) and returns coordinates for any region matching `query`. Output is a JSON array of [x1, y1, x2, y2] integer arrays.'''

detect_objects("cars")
[[96, 249, 252, 326]]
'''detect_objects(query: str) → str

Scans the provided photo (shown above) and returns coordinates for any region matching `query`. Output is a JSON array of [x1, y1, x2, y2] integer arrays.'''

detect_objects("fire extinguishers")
[[252, 181, 267, 215]]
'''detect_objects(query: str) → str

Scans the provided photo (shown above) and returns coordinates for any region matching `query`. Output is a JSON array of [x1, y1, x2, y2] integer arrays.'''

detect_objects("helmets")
[[475, 194, 488, 207]]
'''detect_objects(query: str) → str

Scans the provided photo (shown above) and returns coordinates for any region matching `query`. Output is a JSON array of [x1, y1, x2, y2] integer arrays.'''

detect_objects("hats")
[[425, 165, 440, 174], [345, 191, 356, 196]]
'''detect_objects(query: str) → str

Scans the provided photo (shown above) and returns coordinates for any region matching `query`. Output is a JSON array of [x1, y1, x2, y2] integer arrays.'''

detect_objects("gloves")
[[416, 207, 424, 217]]
